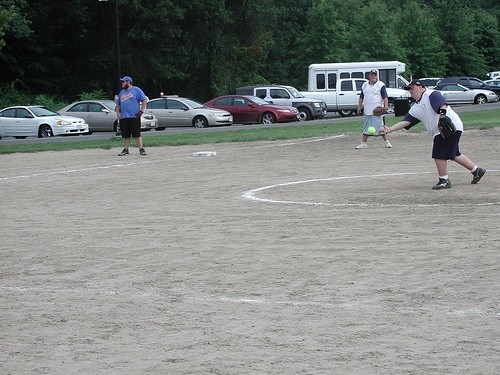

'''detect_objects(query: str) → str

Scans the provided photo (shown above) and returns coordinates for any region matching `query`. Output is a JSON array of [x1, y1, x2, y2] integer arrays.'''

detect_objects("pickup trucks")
[[298, 78, 370, 117]]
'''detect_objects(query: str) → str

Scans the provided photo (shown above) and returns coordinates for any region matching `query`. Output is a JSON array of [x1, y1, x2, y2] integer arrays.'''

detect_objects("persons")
[[115, 76, 150, 156], [377, 78, 487, 189], [356, 69, 394, 150]]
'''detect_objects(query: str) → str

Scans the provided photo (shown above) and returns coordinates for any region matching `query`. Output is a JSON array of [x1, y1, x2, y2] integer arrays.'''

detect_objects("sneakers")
[[355, 143, 368, 149], [471, 167, 486, 184], [140, 148, 147, 155], [118, 148, 129, 156], [432, 177, 452, 189], [384, 141, 392, 148]]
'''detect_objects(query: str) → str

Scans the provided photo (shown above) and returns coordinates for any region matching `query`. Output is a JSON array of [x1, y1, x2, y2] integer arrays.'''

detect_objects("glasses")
[[122, 81, 128, 84]]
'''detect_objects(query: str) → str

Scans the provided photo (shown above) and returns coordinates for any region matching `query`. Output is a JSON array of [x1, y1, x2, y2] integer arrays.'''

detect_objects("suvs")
[[233, 84, 329, 121]]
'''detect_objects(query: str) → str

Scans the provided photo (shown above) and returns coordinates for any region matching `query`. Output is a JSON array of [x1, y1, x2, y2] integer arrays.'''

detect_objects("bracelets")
[[140, 110, 143, 113]]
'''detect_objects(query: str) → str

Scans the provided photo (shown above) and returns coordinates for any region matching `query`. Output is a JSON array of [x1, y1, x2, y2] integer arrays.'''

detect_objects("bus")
[[306, 60, 414, 114]]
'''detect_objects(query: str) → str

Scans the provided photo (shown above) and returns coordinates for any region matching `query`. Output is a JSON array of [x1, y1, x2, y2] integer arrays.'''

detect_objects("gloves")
[[135, 111, 143, 118]]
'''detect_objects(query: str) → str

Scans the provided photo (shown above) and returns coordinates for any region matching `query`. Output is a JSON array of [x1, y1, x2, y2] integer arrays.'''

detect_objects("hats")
[[371, 70, 377, 75], [404, 80, 425, 91], [119, 76, 133, 85]]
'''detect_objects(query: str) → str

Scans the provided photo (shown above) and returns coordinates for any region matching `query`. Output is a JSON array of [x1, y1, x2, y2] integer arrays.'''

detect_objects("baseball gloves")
[[373, 106, 388, 116], [438, 115, 457, 140]]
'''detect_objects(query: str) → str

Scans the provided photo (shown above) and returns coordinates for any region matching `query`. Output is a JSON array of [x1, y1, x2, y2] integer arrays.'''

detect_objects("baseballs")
[[368, 126, 375, 134]]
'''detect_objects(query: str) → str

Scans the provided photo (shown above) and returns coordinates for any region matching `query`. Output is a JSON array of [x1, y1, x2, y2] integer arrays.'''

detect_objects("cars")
[[140, 95, 234, 131], [203, 94, 299, 125], [0, 106, 89, 140], [55, 99, 158, 136], [418, 70, 500, 105]]
